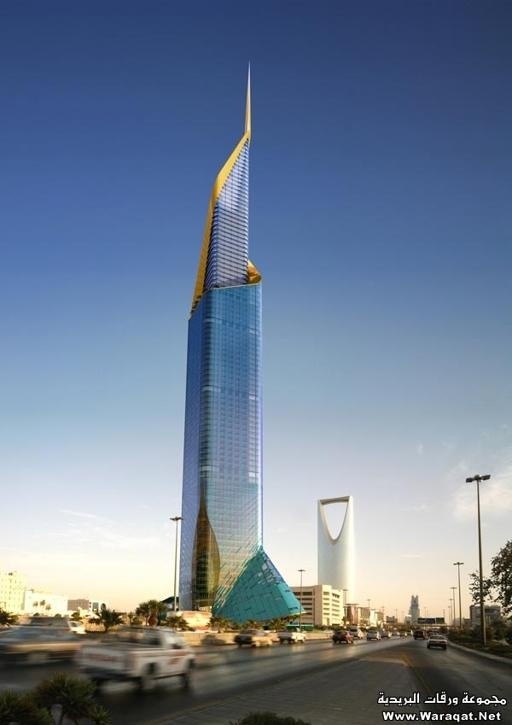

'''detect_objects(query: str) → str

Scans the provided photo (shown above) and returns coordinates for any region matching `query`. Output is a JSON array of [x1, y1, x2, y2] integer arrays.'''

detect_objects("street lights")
[[170, 516, 184, 612], [466, 474, 491, 647], [448, 562, 464, 629], [298, 570, 305, 630]]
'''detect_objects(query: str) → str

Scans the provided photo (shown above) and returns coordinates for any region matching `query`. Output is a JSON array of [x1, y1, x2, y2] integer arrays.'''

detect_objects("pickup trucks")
[[80, 627, 196, 696]]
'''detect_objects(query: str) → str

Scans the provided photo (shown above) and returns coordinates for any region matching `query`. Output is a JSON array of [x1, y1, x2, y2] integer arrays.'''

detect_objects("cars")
[[234, 627, 305, 648], [332, 627, 448, 650], [0, 621, 84, 694]]
